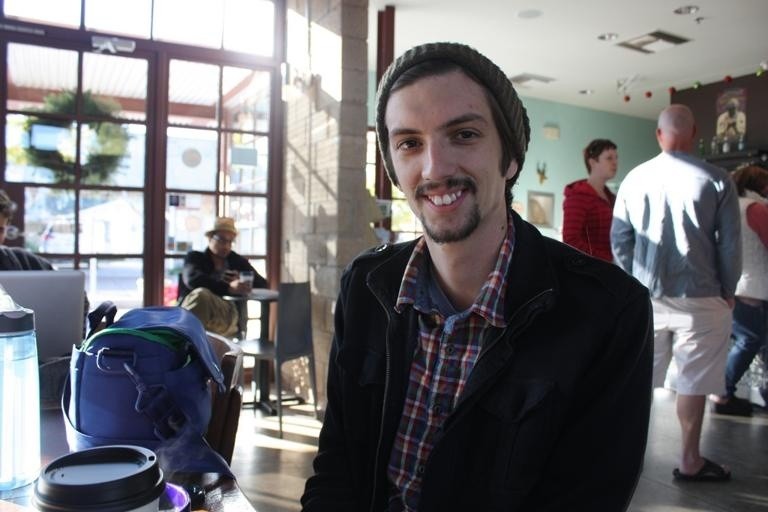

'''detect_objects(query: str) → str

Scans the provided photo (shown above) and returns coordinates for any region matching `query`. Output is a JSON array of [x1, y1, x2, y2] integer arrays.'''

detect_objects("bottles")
[[698, 131, 747, 157], [0, 309, 42, 493]]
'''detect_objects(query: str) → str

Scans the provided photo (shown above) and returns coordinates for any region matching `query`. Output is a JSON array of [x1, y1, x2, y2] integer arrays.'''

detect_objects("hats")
[[375, 43, 531, 188], [205, 217, 239, 235]]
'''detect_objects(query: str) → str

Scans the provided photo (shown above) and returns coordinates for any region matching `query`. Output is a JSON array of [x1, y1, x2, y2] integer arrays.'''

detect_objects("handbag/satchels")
[[61, 300, 224, 477]]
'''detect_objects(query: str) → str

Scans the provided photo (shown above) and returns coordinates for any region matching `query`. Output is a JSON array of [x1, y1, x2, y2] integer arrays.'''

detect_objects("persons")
[[717, 99, 747, 143], [609, 104, 742, 483], [0, 187, 89, 334], [562, 140, 616, 263], [708, 166, 768, 415], [300, 43, 655, 512], [177, 218, 269, 340]]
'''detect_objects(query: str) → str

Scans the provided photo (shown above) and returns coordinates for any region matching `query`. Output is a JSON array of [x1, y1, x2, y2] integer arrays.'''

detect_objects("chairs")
[[239, 279, 320, 438], [205, 330, 243, 465]]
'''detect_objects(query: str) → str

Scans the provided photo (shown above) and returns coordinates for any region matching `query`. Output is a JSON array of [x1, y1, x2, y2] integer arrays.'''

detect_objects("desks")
[[0, 347, 259, 512], [222, 284, 307, 420]]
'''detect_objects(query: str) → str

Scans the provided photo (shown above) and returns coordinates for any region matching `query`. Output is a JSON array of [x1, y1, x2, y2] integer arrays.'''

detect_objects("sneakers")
[[709, 394, 752, 414]]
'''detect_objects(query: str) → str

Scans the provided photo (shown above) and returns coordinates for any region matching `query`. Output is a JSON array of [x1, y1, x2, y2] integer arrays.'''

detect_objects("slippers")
[[673, 458, 730, 481]]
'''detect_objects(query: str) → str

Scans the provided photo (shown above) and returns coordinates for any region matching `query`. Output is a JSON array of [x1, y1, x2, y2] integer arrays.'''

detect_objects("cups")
[[239, 270, 254, 295], [160, 480, 192, 512], [31, 445, 162, 512]]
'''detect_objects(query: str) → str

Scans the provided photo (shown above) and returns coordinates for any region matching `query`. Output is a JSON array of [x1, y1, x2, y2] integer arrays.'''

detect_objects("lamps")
[[280, 59, 316, 106]]
